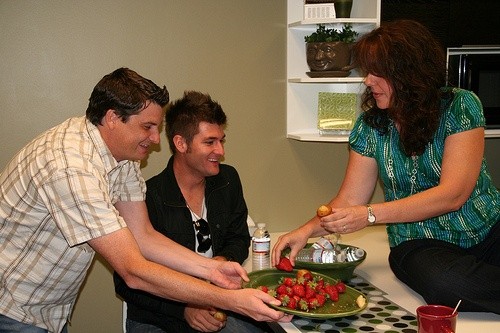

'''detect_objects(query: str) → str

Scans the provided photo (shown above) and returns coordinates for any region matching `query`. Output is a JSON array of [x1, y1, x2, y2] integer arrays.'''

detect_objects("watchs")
[[366, 204, 377, 225]]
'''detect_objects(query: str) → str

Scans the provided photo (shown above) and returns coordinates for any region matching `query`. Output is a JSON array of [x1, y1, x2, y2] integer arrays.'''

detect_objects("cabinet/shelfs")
[[286, 0, 381, 142]]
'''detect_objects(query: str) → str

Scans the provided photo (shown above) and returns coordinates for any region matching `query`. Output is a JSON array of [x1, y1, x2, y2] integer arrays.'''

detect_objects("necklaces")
[[184, 180, 205, 220], [387, 122, 418, 200]]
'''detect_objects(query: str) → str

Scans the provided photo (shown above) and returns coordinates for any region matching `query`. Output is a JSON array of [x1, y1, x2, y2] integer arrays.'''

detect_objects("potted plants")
[[304, 22, 361, 71]]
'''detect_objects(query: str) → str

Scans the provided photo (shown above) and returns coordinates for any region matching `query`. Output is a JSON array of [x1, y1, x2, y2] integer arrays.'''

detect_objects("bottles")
[[284, 249, 345, 264], [309, 232, 342, 250], [252, 223, 271, 270], [345, 247, 364, 262]]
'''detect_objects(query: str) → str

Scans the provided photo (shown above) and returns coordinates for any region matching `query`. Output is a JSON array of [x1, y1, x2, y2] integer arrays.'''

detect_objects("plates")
[[242, 268, 368, 319]]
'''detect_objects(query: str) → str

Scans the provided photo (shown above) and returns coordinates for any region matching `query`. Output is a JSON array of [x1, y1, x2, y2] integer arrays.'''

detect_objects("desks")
[[238, 224, 500, 333]]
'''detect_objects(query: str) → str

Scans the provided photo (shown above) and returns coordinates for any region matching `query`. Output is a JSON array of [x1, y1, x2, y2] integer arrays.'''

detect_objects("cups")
[[334, 0, 352, 18], [416, 305, 459, 333]]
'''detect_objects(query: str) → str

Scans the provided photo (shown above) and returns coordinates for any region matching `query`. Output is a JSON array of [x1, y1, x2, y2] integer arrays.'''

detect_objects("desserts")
[[213, 311, 226, 327], [317, 204, 333, 218]]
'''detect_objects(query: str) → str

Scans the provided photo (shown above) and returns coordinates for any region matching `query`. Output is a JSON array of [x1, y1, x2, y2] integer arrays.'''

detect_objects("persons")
[[0, 67, 293, 333], [269, 20, 500, 316], [113, 90, 276, 333]]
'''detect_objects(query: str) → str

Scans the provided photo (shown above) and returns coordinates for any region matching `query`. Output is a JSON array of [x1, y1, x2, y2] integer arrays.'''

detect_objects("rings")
[[212, 312, 221, 318], [343, 225, 346, 232]]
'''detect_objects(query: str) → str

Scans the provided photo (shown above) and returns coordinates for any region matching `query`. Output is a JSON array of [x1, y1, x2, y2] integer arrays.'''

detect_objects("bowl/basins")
[[282, 243, 367, 284]]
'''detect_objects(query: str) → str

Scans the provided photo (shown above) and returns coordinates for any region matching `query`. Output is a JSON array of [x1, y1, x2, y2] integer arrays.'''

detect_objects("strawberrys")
[[275, 257, 293, 271], [256, 272, 345, 311]]
